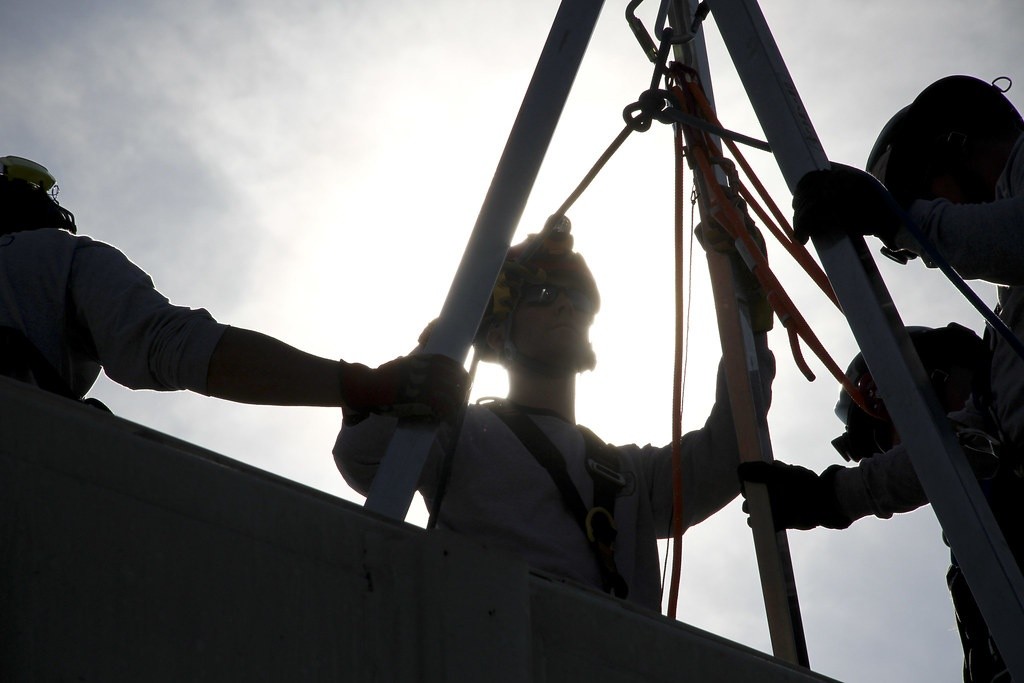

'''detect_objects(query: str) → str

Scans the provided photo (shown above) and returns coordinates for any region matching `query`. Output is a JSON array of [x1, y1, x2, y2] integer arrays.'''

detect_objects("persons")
[[332, 230, 778, 616], [794, 76, 1024, 473], [1, 153, 471, 419], [735, 322, 1023, 682]]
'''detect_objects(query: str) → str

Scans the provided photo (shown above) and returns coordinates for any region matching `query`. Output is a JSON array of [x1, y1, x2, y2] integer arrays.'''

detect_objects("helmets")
[[476, 242, 602, 365], [834, 323, 983, 461], [867, 75, 1023, 262], [1, 154, 76, 233]]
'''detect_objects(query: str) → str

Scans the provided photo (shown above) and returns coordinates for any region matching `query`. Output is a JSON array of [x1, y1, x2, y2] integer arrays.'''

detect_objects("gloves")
[[339, 351, 471, 429], [694, 187, 774, 332], [790, 160, 893, 246], [736, 462, 850, 537]]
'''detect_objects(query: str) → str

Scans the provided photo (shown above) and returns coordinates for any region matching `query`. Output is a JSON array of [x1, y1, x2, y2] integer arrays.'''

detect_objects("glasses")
[[521, 280, 592, 314]]
